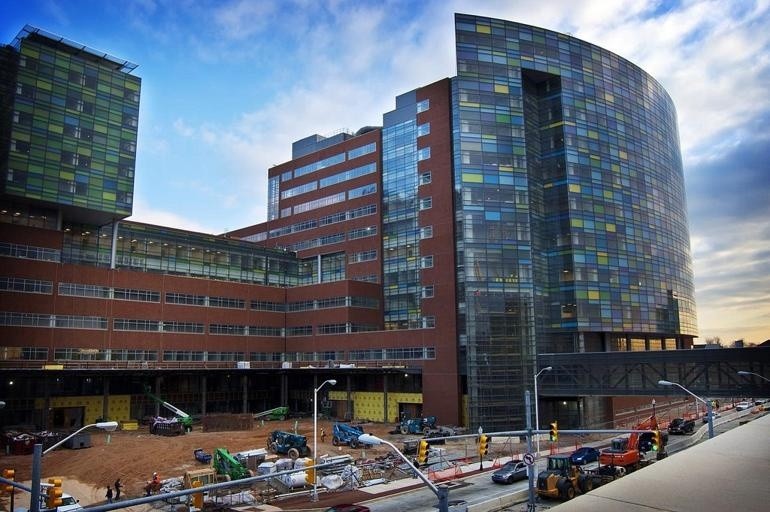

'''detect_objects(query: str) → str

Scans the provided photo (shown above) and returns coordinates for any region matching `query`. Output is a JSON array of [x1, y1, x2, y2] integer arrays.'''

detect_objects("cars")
[[324, 503, 369, 512], [53, 492, 86, 511], [703, 411, 721, 423], [569, 446, 600, 465], [736, 397, 770, 413], [667, 417, 696, 435], [491, 458, 528, 484], [639, 430, 668, 450]]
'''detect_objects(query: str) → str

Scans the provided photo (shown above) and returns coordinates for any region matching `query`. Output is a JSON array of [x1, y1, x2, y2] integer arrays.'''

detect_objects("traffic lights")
[[419, 439, 429, 464], [1, 467, 15, 496], [305, 458, 316, 485], [651, 431, 659, 451], [47, 477, 62, 509], [549, 420, 558, 441], [479, 434, 489, 458]]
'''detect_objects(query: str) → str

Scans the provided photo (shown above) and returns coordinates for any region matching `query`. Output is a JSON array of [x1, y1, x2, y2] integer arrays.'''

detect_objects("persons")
[[153, 472, 159, 484], [320, 428, 325, 443], [115, 479, 120, 499], [105, 485, 113, 504]]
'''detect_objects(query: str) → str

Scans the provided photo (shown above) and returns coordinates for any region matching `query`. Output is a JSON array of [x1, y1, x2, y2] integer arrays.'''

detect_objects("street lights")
[[29, 421, 118, 511], [658, 380, 714, 439], [311, 379, 338, 501], [531, 366, 555, 454], [738, 370, 770, 384], [357, 432, 448, 511]]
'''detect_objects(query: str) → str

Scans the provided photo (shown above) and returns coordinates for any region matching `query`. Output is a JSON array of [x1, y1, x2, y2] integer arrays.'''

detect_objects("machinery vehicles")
[[397, 414, 439, 436], [331, 421, 370, 448], [213, 447, 254, 483], [598, 415, 663, 477], [138, 382, 194, 437], [265, 429, 310, 459], [535, 456, 602, 502], [251, 404, 290, 421]]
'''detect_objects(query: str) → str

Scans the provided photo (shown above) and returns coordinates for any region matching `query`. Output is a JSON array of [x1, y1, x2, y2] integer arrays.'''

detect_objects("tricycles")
[[193, 448, 212, 465]]
